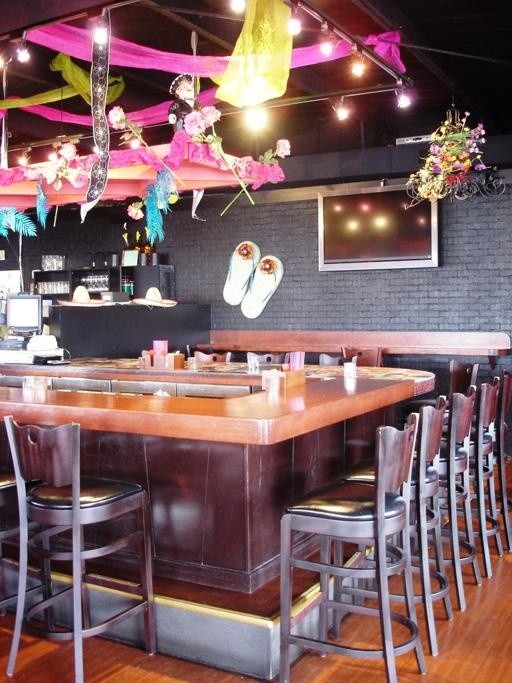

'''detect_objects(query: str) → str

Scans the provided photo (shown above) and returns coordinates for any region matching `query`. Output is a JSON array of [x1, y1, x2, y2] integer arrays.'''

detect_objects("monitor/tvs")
[[317, 184, 439, 271]]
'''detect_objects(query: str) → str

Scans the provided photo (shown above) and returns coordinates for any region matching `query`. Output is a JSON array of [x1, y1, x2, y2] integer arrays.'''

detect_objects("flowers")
[[183, 106, 256, 206], [108, 107, 185, 189], [20, 143, 86, 227], [220, 139, 290, 214], [404, 112, 486, 209]]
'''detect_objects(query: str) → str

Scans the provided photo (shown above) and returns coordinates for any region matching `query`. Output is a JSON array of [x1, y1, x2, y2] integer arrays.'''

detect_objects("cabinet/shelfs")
[[34, 265, 176, 323]]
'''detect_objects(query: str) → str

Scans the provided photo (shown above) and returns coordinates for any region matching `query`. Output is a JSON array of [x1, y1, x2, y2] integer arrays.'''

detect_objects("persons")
[[168, 72, 207, 222]]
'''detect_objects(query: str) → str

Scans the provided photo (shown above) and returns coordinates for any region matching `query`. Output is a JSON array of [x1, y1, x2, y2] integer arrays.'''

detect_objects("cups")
[[111, 253, 159, 267], [344, 362, 356, 379], [343, 379, 357, 395], [165, 353, 202, 371]]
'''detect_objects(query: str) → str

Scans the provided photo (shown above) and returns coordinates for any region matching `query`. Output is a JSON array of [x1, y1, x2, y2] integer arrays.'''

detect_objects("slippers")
[[241, 255, 283, 318], [223, 241, 260, 305]]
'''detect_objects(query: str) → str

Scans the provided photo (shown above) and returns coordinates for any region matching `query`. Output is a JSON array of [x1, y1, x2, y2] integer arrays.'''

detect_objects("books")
[[0, 350, 46, 364]]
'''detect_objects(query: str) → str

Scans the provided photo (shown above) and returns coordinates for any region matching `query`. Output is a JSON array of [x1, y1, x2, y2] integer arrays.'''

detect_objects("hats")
[[135, 287, 177, 308], [57, 285, 104, 307]]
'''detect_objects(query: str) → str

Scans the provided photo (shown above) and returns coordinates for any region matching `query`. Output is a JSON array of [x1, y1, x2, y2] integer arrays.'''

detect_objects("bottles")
[[122, 276, 134, 296]]
